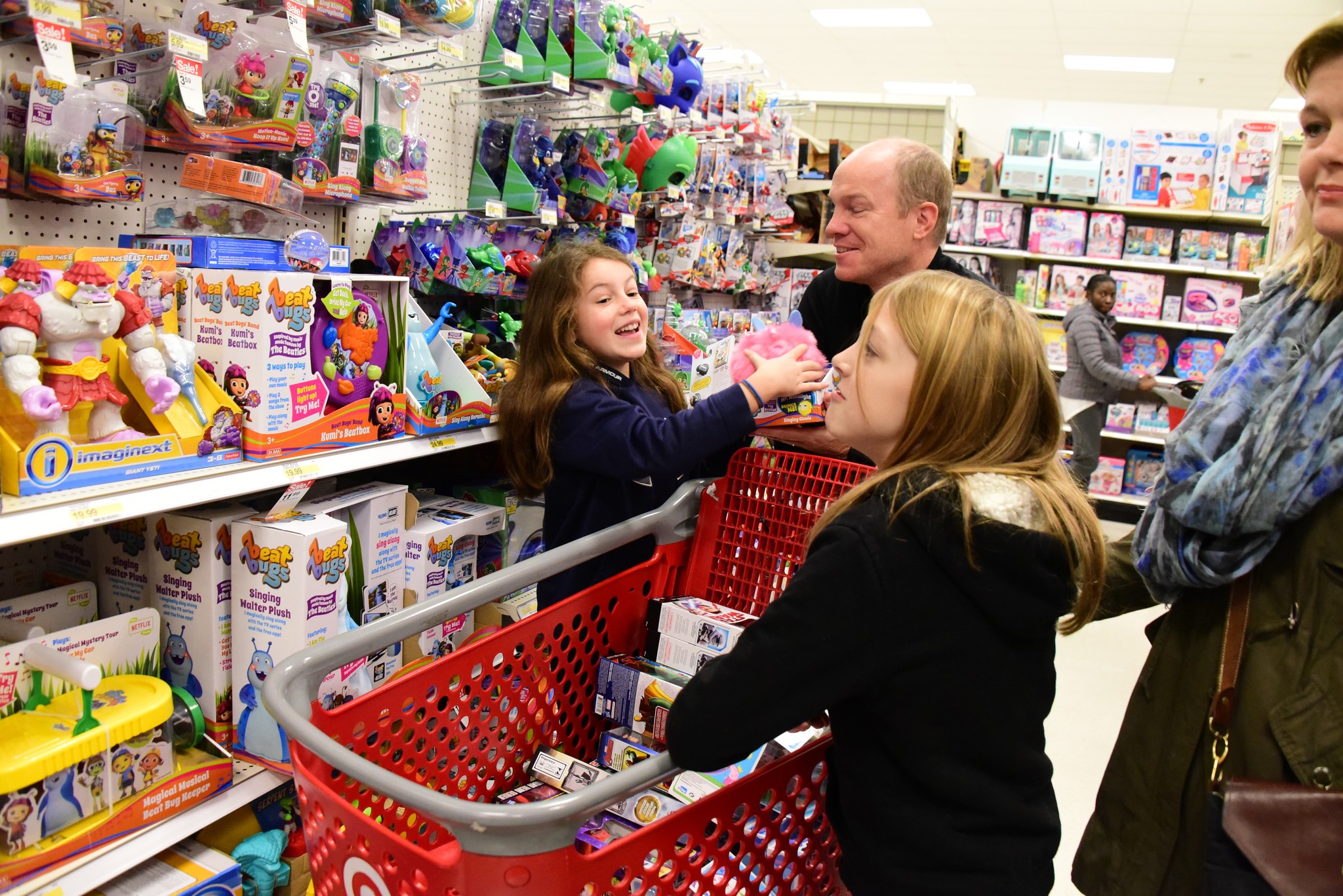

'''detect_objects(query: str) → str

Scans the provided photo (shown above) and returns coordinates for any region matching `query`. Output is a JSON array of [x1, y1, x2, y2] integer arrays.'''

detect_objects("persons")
[[1055, 274, 1086, 298], [1094, 22, 1343, 896], [501, 239, 827, 764], [669, 269, 1106, 896], [1186, 175, 1210, 210], [1157, 173, 1173, 207], [752, 137, 999, 496], [1058, 275, 1159, 493], [956, 199, 1022, 277]]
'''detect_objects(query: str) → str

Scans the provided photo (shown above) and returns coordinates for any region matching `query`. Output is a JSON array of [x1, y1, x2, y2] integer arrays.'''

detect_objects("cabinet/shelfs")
[[0, 423, 504, 896], [942, 190, 1268, 505], [763, 180, 837, 263]]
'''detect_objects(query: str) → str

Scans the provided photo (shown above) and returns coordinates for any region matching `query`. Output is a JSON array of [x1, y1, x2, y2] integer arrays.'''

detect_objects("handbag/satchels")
[[1205, 782, 1343, 896]]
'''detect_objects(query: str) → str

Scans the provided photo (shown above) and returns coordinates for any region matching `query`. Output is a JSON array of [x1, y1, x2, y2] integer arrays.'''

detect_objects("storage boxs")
[[93, 234, 830, 896], [944, 121, 1280, 496]]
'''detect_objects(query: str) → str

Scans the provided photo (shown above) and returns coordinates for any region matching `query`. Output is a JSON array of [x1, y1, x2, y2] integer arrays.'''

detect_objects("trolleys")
[[259, 448, 885, 896]]
